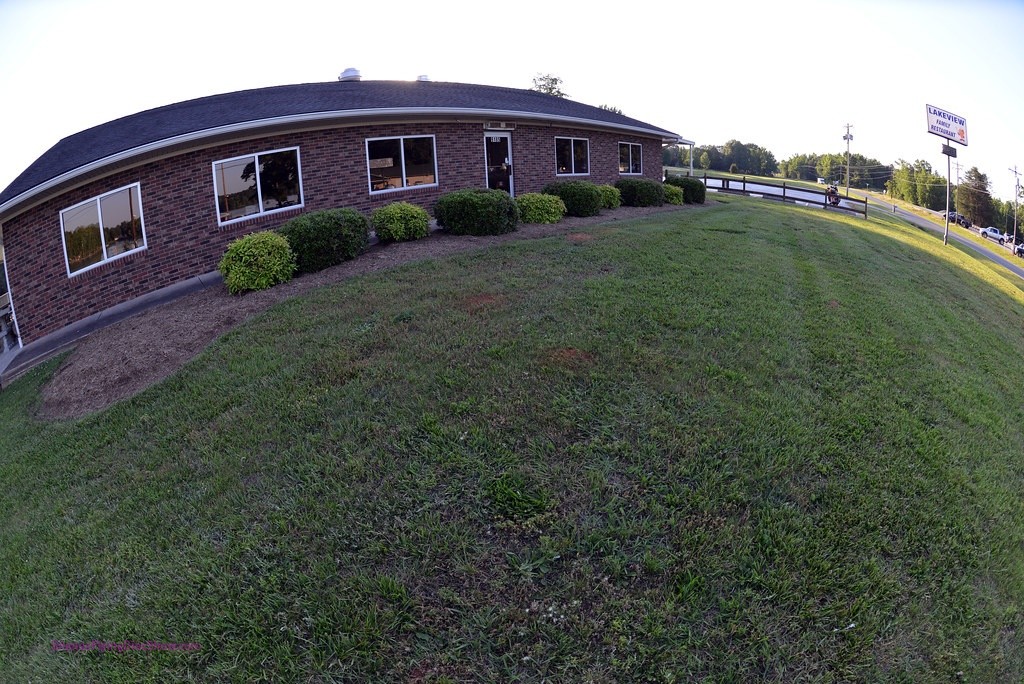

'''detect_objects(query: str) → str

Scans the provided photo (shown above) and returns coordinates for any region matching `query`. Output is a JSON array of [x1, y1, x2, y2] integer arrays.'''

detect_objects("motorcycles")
[[827, 181, 841, 206]]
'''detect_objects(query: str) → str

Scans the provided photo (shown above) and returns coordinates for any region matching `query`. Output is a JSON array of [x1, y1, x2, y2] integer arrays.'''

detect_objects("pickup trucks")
[[1004, 232, 1024, 258], [979, 227, 1006, 245]]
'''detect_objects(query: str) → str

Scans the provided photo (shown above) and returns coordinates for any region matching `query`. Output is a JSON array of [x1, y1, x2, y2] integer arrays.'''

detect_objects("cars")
[[943, 212, 972, 228]]
